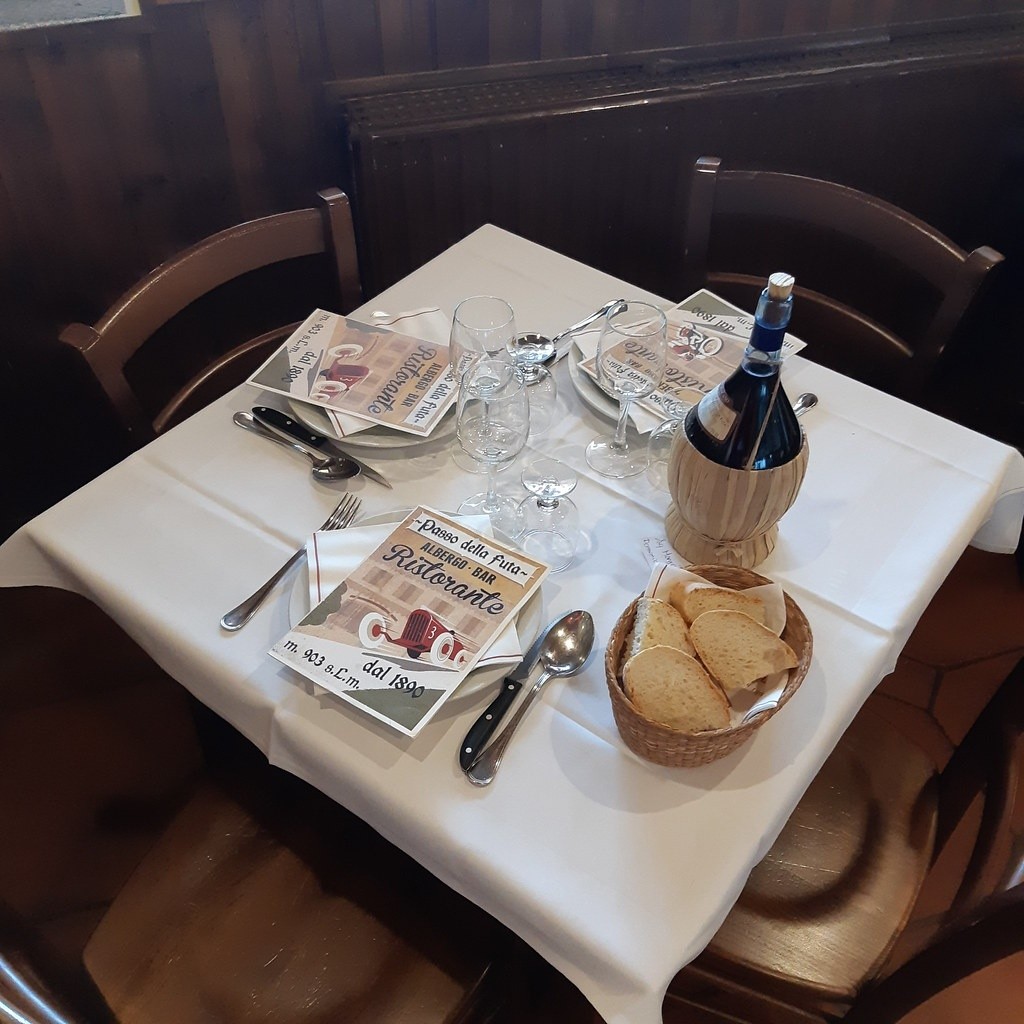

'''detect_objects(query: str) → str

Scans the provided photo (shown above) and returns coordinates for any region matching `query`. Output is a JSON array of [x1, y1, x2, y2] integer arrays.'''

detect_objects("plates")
[[288, 397, 459, 451], [568, 340, 652, 433], [288, 509, 542, 702]]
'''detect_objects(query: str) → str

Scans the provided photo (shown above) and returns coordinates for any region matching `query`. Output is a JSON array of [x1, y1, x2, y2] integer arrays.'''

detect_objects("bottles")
[[664, 270, 810, 566]]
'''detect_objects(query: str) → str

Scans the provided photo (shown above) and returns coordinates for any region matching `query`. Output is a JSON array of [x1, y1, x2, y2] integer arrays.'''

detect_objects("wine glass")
[[448, 294, 521, 475], [513, 457, 581, 572], [585, 300, 667, 479], [455, 360, 532, 539], [644, 387, 707, 495], [504, 330, 557, 437]]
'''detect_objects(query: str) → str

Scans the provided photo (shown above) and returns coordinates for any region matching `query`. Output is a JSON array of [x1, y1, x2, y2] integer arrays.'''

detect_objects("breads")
[[617, 582, 800, 727]]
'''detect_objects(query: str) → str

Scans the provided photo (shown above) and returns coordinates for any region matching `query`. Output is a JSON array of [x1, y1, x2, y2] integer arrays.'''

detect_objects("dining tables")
[[1, 217, 1021, 1024]]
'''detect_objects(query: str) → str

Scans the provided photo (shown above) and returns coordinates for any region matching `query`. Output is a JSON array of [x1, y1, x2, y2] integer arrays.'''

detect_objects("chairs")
[[674, 650, 1021, 1024], [662, 153, 1010, 409], [57, 188, 368, 454], [1, 738, 512, 1024]]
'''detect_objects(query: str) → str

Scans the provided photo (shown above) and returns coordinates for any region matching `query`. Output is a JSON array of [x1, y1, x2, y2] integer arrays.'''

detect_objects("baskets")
[[605, 563, 814, 769]]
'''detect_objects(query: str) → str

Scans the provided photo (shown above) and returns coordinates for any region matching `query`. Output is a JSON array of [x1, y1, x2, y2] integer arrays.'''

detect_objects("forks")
[[219, 489, 363, 633]]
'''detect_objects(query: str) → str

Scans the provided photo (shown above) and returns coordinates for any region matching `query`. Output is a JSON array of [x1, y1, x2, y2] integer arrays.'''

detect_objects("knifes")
[[457, 609, 575, 775], [250, 402, 394, 491]]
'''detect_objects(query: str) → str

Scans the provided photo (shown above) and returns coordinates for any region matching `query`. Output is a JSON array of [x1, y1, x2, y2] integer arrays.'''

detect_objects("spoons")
[[230, 409, 362, 484], [514, 297, 628, 365], [464, 609, 594, 786]]
